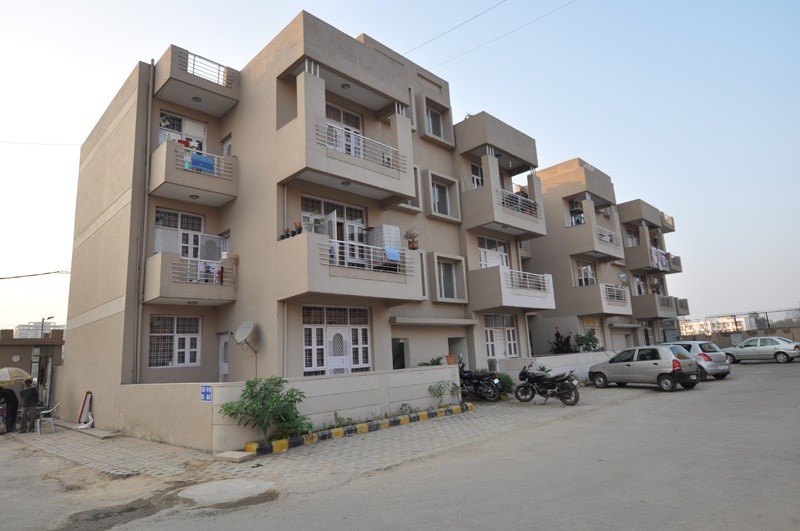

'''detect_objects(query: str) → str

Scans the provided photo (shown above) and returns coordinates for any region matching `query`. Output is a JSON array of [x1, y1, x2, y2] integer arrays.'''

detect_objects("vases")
[[280, 227, 302, 239], [445, 354, 455, 364]]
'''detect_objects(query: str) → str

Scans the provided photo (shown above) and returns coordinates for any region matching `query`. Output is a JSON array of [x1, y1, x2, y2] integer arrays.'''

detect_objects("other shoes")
[[29, 429, 36, 432], [19, 430, 27, 433]]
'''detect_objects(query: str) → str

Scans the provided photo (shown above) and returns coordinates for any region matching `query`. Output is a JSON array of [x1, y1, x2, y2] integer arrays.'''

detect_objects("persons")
[[0, 386, 19, 432], [18, 379, 39, 433]]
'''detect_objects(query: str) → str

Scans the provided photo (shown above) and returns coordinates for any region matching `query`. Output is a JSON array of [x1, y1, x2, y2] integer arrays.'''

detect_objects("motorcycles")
[[458, 352, 502, 401], [514, 361, 580, 405]]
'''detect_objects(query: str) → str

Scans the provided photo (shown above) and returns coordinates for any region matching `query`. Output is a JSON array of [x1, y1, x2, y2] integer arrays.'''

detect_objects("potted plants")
[[403, 227, 418, 249]]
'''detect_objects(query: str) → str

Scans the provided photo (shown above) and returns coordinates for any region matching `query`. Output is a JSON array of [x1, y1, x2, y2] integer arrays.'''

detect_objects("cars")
[[721, 337, 800, 364], [588, 345, 700, 392], [661, 341, 730, 382]]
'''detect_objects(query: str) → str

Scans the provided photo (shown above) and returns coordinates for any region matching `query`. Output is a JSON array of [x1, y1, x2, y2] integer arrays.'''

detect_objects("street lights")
[[37, 315, 55, 390]]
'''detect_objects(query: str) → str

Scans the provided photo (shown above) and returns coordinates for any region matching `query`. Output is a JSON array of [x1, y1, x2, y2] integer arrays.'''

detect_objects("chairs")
[[36, 402, 60, 435]]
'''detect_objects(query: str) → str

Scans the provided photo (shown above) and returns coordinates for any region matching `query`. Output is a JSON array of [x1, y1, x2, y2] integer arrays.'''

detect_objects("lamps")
[[192, 96, 201, 102], [341, 83, 350, 89], [495, 153, 502, 157], [342, 181, 351, 185], [189, 194, 199, 199]]
